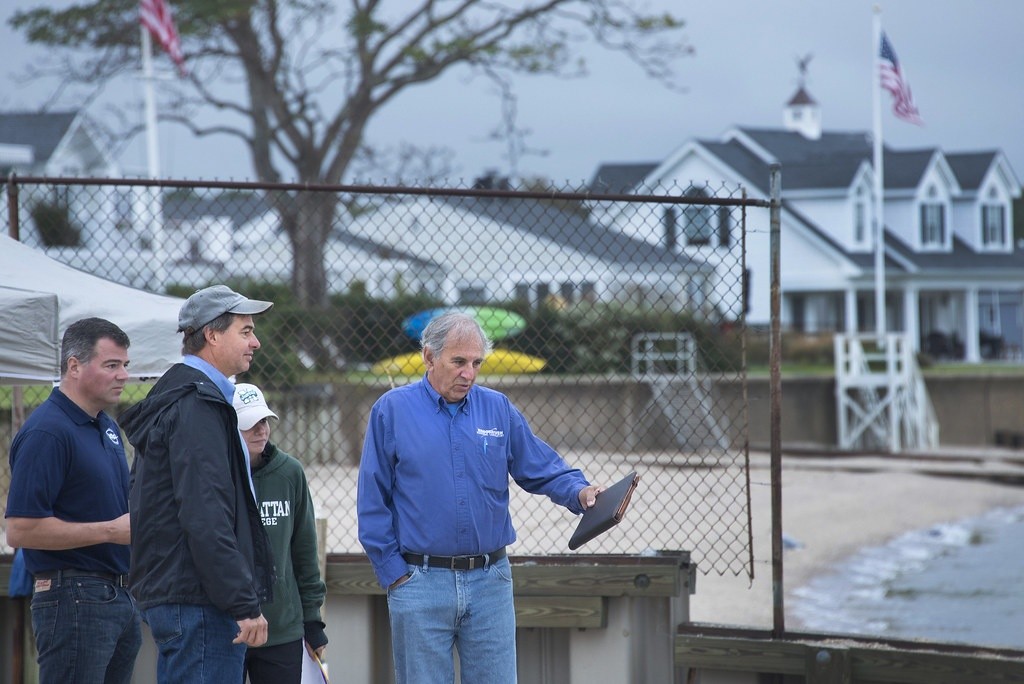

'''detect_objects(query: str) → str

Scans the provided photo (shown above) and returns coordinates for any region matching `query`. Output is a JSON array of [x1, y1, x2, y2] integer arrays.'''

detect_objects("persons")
[[5, 285, 329, 684], [356, 314, 608, 684]]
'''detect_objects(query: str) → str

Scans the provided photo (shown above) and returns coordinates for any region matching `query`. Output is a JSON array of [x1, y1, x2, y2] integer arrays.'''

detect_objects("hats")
[[232, 383, 279, 432], [178, 285, 273, 332]]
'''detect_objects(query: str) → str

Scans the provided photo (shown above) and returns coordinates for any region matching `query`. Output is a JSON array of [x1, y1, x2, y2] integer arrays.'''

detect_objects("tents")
[[0, 233, 187, 383]]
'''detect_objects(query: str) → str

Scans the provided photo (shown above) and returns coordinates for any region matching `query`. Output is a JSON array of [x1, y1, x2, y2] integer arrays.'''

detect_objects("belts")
[[404, 546, 507, 571], [35, 569, 126, 587]]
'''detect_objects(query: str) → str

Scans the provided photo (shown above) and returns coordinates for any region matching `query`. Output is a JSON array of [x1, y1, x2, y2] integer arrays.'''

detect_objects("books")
[[568, 472, 640, 550]]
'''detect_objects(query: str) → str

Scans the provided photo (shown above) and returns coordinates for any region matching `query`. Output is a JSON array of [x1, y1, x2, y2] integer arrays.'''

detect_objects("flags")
[[139, 0, 187, 77]]
